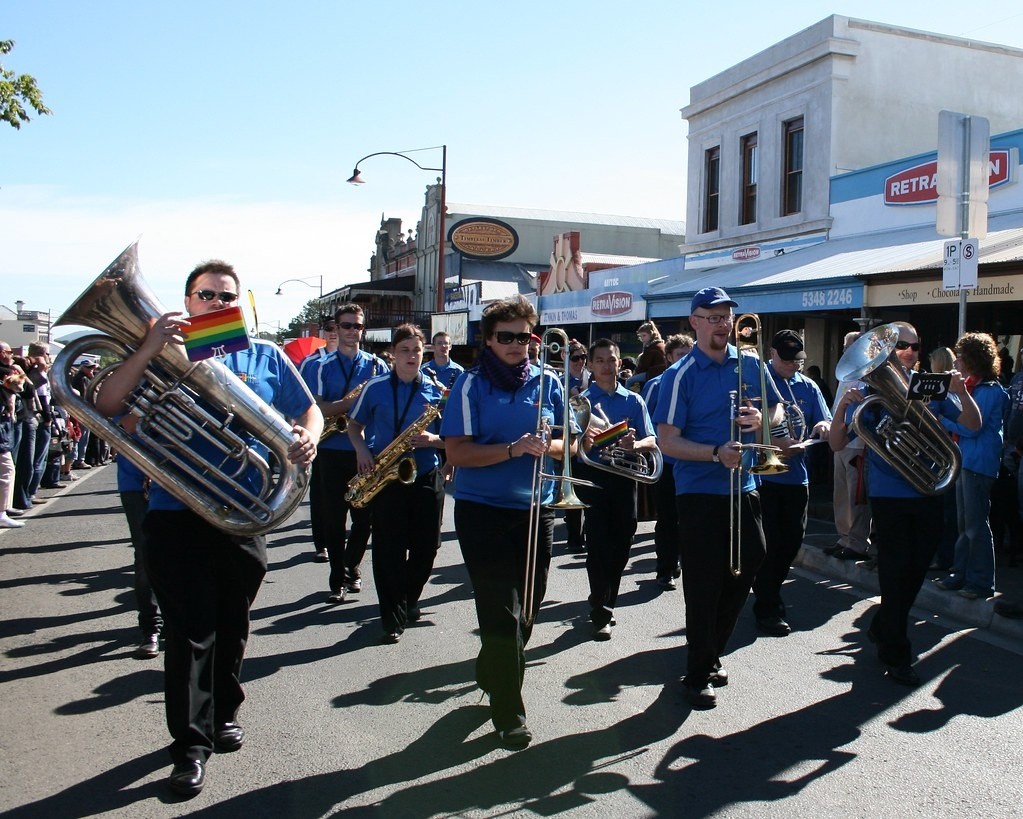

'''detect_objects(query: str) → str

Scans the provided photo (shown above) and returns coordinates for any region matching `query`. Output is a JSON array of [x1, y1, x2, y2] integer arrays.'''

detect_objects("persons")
[[1, 287, 1023, 745], [95, 259, 324, 795]]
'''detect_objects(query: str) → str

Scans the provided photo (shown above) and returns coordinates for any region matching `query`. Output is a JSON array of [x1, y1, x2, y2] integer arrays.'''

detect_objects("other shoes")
[[475, 645, 491, 695], [706, 671, 728, 688], [756, 614, 792, 634], [822, 541, 1023, 620], [776, 597, 786, 615], [0, 449, 116, 528], [681, 688, 715, 708], [307, 519, 684, 644], [492, 713, 532, 744]]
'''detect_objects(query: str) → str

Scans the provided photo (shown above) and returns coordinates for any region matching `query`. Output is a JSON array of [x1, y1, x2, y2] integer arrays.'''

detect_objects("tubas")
[[49, 239, 312, 537], [563, 394, 663, 483], [835, 322, 964, 497]]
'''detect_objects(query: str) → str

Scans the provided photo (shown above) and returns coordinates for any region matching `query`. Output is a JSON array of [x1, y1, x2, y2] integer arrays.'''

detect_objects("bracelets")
[[508, 441, 513, 459]]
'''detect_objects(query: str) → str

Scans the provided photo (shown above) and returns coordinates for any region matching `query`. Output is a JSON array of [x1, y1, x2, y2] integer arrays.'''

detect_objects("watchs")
[[712, 445, 721, 463]]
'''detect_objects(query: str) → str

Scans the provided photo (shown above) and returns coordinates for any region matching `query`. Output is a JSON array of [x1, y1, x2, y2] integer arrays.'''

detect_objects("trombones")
[[522, 328, 600, 628], [730, 314, 790, 575]]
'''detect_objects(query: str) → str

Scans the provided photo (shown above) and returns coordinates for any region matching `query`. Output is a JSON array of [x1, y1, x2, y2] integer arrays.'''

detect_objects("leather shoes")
[[138, 634, 160, 657], [886, 663, 920, 684], [867, 629, 903, 664], [213, 715, 245, 754], [172, 756, 204, 792]]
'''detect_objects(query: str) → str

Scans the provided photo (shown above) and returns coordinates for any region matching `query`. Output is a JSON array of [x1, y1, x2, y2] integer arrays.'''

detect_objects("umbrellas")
[[284, 336, 327, 365]]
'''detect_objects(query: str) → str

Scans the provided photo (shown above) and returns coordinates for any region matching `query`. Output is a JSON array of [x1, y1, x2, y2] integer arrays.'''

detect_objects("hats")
[[691, 287, 739, 315], [81, 360, 96, 367], [530, 333, 542, 344], [772, 328, 807, 360]]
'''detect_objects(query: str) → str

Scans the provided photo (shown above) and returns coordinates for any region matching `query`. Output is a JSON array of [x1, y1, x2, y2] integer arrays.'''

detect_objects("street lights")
[[274, 276, 324, 334], [345, 144, 447, 313]]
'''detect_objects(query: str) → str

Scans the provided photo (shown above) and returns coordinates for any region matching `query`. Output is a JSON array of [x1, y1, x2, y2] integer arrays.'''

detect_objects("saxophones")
[[345, 372, 461, 511], [319, 358, 380, 447]]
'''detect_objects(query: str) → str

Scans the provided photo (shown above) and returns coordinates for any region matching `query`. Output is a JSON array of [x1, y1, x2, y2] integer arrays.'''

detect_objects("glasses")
[[339, 322, 364, 330], [693, 314, 736, 324], [569, 354, 588, 362], [896, 341, 920, 352], [190, 290, 238, 304], [490, 330, 531, 345], [325, 326, 337, 332], [954, 351, 963, 358], [4, 350, 14, 355], [779, 357, 804, 365]]
